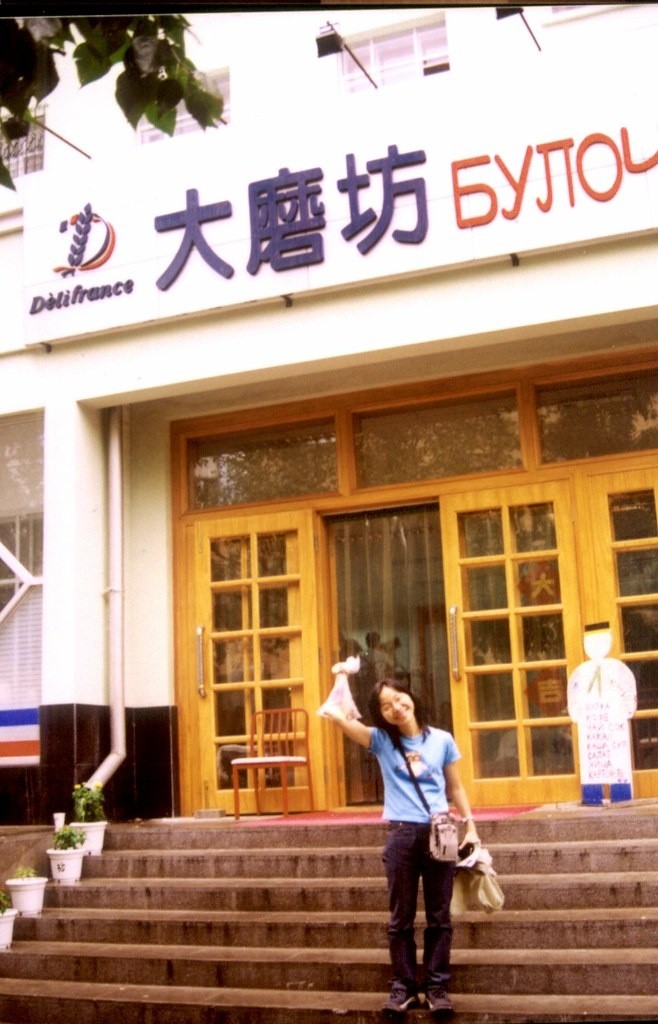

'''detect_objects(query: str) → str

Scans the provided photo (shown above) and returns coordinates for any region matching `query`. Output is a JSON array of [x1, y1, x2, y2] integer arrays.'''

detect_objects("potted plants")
[[46, 826, 87, 884], [5, 866, 48, 917], [0, 893, 17, 950], [69, 781, 107, 857]]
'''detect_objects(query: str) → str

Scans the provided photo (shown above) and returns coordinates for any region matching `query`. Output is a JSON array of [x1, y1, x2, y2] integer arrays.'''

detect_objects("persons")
[[355, 631, 395, 683], [331, 662, 482, 1014]]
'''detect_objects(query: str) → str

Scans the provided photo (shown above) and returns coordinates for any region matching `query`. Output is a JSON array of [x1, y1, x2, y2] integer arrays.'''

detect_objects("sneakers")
[[423, 988, 452, 1012], [380, 988, 418, 1012]]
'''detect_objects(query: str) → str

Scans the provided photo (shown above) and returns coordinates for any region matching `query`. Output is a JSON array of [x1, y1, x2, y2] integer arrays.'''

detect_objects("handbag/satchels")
[[448, 843, 506, 914], [316, 656, 363, 722], [430, 814, 460, 863]]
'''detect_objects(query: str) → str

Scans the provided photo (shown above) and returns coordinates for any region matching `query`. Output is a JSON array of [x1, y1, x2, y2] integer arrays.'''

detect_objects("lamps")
[[4, 110, 91, 160], [495, 6, 540, 52], [316, 21, 380, 88]]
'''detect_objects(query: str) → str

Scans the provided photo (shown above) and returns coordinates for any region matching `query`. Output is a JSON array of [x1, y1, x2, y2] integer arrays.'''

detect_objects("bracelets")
[[461, 816, 474, 824]]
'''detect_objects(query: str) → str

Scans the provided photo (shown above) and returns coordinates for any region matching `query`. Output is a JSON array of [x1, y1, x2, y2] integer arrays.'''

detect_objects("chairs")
[[230, 709, 314, 820]]
